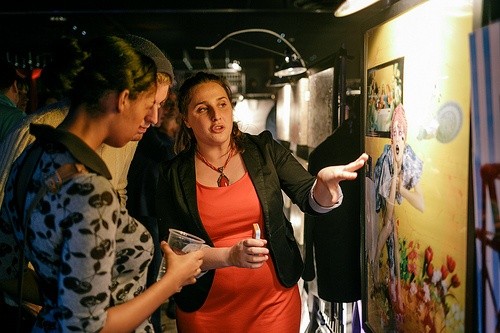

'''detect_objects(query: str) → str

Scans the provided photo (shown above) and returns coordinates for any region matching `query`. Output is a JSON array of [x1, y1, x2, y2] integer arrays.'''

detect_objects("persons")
[[158, 71, 368, 333], [0, 64, 27, 143], [0, 34, 175, 333], [0, 48, 205, 333]]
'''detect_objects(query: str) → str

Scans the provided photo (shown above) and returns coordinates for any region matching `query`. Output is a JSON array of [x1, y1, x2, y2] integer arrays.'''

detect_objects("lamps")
[[266, 46, 308, 87]]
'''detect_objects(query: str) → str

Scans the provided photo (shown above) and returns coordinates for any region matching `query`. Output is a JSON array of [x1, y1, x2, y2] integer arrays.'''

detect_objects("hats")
[[119, 33, 174, 86]]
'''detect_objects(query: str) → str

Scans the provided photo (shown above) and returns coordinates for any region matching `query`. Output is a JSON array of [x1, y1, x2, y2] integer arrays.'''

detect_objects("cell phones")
[[252, 223, 261, 256]]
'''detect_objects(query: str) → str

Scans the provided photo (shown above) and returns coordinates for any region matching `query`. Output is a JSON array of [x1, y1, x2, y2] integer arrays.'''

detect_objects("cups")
[[157, 229, 205, 285]]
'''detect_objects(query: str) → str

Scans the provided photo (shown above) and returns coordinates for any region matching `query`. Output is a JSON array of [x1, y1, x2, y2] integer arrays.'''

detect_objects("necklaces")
[[195, 144, 239, 187]]
